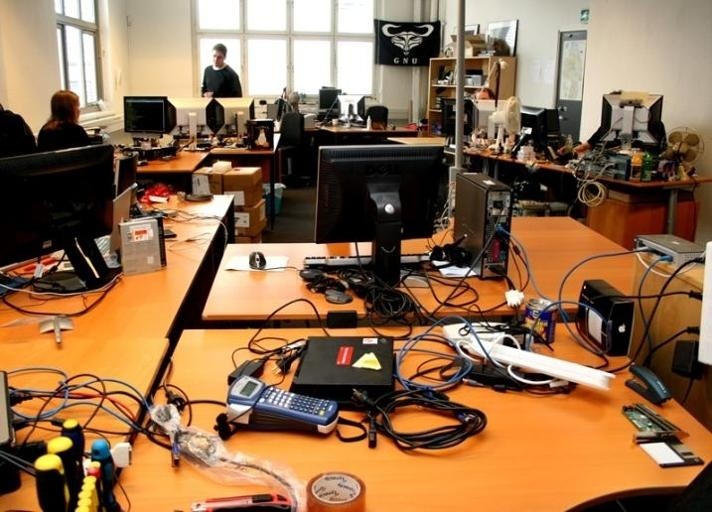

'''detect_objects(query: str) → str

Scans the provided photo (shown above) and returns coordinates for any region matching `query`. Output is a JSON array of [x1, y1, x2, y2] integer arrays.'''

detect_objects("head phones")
[[428, 240, 473, 271]]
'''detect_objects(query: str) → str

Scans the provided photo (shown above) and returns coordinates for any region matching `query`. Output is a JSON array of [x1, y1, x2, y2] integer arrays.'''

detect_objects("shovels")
[[39, 313, 74, 344]]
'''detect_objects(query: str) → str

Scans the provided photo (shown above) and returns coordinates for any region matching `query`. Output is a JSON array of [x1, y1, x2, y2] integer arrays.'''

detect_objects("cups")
[[331, 119, 339, 127]]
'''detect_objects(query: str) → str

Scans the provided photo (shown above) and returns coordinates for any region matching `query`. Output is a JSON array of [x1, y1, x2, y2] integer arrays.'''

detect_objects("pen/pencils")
[[635, 404, 680, 432]]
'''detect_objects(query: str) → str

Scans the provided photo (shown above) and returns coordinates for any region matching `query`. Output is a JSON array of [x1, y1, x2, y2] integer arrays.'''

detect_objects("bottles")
[[366, 115, 372, 131], [629, 148, 652, 182], [564, 134, 573, 152]]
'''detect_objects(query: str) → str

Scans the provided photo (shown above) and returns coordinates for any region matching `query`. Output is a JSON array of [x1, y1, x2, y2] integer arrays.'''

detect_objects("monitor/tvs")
[[600, 92, 664, 155], [336, 94, 365, 123], [319, 87, 342, 112], [0, 143, 115, 294], [441, 99, 561, 145], [123, 96, 254, 138], [315, 145, 445, 288]]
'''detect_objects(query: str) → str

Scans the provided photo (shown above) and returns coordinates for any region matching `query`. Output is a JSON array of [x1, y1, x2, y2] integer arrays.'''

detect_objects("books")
[[247, 119, 273, 149]]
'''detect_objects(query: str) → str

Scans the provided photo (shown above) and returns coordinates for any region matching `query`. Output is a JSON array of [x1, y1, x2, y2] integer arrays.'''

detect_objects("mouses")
[[250, 252, 266, 268]]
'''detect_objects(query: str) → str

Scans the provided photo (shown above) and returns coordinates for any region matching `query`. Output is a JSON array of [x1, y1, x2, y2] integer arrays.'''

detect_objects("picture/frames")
[[484, 17, 521, 60], [450, 22, 481, 48]]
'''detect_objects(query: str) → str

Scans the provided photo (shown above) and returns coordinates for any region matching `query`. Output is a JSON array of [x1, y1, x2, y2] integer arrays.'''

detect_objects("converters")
[[228, 359, 263, 383]]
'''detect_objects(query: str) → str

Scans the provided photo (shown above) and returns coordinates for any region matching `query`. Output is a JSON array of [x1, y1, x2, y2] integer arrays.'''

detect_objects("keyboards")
[[304, 256, 431, 265]]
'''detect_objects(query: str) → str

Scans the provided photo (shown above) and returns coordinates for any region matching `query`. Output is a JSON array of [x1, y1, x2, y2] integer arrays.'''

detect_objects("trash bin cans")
[[263, 183, 284, 215]]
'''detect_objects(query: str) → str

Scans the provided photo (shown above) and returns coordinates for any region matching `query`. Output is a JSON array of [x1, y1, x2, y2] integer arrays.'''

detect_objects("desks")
[[202, 215, 637, 321], [119, 126, 711, 244], [3, 195, 234, 512], [108, 323, 710, 511]]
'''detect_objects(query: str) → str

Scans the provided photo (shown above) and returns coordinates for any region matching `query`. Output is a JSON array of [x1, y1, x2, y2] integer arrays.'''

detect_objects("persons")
[[37, 90, 90, 151], [0, 105, 38, 160], [471, 89, 493, 99], [557, 90, 666, 156], [201, 42, 242, 97]]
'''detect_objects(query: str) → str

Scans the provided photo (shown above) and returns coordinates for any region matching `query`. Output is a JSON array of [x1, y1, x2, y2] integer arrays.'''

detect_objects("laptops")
[[95, 187, 133, 254]]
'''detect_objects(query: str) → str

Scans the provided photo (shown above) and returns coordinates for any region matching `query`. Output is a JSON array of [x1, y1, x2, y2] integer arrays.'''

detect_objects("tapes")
[[306, 472, 366, 512]]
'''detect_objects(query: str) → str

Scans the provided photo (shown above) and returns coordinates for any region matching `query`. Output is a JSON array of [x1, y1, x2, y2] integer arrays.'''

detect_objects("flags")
[[376, 19, 440, 67]]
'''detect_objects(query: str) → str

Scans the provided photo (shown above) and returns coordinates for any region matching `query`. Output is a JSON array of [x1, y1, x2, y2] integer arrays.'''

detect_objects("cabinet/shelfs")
[[629, 248, 710, 431], [424, 53, 519, 140]]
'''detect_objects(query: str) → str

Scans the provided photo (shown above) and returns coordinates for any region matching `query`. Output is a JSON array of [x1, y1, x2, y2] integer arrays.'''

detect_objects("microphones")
[[451, 229, 468, 247]]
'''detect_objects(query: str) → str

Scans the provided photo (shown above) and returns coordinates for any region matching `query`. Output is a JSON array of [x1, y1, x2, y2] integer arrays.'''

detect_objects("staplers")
[[191, 493, 292, 512], [625, 362, 672, 405]]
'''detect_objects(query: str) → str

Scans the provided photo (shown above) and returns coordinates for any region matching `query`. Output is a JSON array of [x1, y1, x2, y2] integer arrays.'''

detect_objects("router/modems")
[[635, 233, 705, 263]]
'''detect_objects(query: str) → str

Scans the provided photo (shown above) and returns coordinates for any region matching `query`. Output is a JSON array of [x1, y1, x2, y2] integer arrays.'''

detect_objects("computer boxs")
[[454, 172, 514, 279]]
